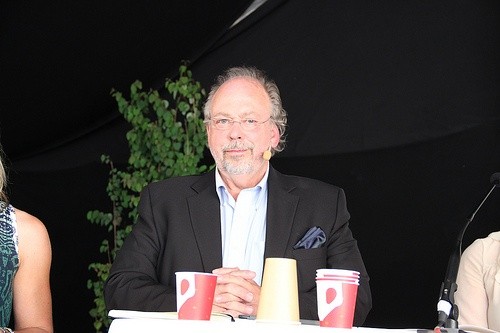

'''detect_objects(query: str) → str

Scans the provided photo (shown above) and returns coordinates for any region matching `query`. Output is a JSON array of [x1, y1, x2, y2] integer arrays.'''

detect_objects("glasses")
[[205, 115, 271, 131]]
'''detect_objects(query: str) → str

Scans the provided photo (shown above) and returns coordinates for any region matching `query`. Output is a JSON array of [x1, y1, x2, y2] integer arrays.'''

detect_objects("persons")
[[456, 230, 500, 333], [103, 66, 374, 327], [0, 144, 54, 333]]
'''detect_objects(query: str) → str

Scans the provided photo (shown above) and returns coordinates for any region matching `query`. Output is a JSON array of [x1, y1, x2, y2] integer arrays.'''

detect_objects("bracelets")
[[1, 326, 13, 333]]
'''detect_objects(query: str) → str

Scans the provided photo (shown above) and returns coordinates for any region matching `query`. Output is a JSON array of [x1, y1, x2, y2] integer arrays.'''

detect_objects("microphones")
[[263, 146, 271, 161], [433, 173, 500, 333]]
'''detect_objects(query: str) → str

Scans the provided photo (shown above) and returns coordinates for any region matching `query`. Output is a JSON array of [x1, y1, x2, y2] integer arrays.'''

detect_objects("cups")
[[315, 268, 360, 329], [174, 271, 218, 320], [254, 257, 302, 324]]
